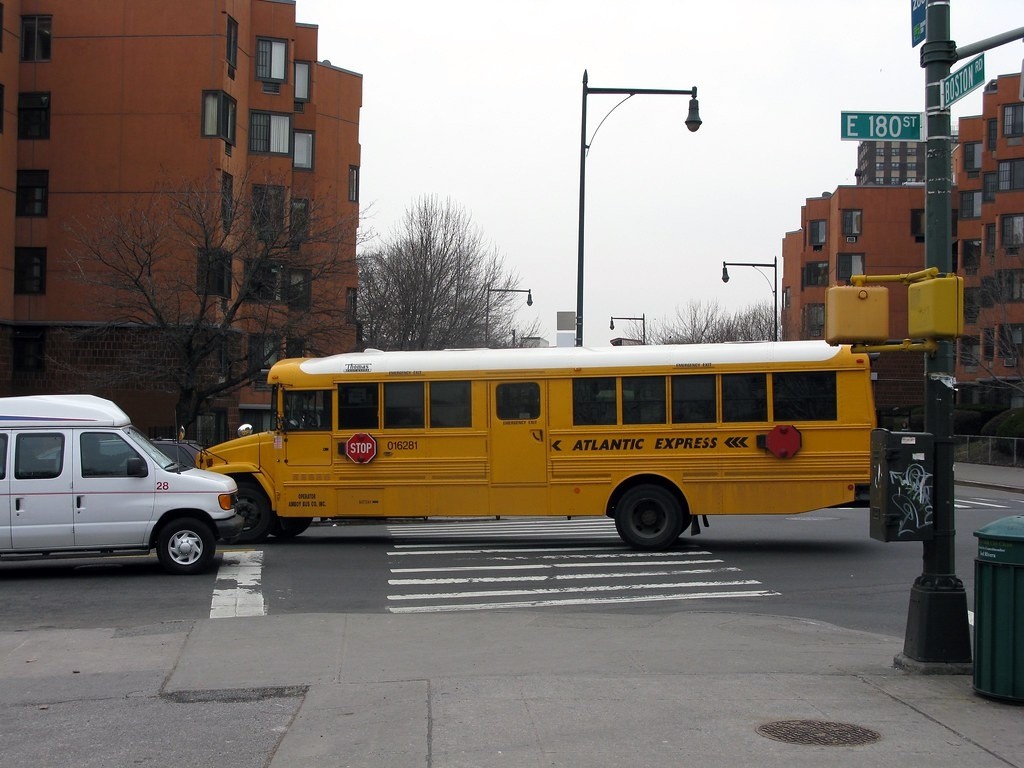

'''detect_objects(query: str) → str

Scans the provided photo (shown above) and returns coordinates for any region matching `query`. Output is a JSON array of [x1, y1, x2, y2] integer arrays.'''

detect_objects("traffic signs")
[[841, 110, 924, 142], [940, 51, 986, 110]]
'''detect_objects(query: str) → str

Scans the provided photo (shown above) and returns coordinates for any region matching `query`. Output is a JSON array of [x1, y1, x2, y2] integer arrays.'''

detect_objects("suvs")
[[32, 436, 201, 472]]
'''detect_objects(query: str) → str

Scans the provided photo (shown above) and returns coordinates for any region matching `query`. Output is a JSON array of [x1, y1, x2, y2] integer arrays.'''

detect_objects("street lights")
[[722, 256, 777, 340], [609, 314, 645, 346], [574, 68, 701, 348], [485, 283, 533, 348]]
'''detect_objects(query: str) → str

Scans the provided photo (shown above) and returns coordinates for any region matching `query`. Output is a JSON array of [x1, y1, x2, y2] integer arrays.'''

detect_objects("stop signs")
[[347, 433, 376, 463]]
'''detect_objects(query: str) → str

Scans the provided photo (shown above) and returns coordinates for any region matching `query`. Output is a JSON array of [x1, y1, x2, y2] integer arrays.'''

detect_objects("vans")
[[0, 393, 242, 575]]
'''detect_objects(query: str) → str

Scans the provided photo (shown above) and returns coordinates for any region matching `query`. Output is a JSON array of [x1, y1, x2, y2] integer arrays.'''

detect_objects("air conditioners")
[[847, 237, 855, 243], [1004, 358, 1018, 367]]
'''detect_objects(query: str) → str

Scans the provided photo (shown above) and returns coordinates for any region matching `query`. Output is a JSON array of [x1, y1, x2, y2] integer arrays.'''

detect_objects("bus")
[[176, 339, 877, 550]]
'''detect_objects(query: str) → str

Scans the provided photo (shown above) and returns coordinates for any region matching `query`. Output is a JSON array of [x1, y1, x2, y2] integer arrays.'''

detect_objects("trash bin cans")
[[972, 516, 1024, 705]]
